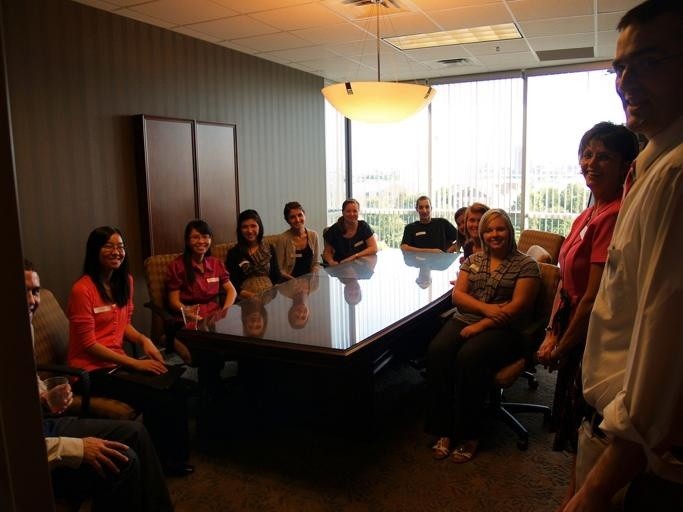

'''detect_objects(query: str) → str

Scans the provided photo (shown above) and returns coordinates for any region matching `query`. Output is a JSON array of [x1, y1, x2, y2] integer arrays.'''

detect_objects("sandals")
[[452, 440, 479, 463], [432, 437, 448, 460]]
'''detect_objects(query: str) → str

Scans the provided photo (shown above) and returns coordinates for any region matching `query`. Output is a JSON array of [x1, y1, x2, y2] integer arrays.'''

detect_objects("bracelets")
[[545, 326, 553, 331], [356, 253, 359, 259]]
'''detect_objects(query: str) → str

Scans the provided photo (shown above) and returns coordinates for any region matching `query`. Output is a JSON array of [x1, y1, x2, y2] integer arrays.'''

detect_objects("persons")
[[182, 308, 227, 333], [323, 198, 377, 266], [24, 259, 174, 512], [164, 220, 238, 327], [560, 0, 682, 512], [324, 255, 377, 306], [278, 270, 320, 329], [67, 226, 195, 479], [275, 202, 320, 283], [400, 196, 541, 464], [537, 121, 639, 457], [226, 209, 283, 304], [235, 287, 278, 338]]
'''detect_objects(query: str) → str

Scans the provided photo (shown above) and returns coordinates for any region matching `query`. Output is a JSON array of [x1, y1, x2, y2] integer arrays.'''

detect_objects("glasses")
[[102, 244, 128, 253]]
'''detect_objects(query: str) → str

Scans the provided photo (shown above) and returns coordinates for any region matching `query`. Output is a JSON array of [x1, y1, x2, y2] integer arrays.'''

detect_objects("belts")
[[583, 403, 602, 430]]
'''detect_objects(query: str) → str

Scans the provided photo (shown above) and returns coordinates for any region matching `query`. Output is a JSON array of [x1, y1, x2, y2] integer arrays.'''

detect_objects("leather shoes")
[[165, 463, 193, 477]]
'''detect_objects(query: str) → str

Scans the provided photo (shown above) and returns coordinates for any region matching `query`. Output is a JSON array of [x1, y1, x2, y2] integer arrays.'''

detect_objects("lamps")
[[321, 0, 436, 123]]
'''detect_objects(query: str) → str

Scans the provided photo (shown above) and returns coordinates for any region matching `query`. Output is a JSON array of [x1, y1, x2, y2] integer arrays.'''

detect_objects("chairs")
[[474, 262, 562, 451], [33, 289, 138, 420], [516, 230, 566, 265], [143, 253, 186, 353], [210, 234, 282, 265], [523, 244, 552, 391]]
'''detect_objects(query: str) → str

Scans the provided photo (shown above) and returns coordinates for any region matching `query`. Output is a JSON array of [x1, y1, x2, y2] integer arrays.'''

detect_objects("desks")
[[179, 247, 475, 490]]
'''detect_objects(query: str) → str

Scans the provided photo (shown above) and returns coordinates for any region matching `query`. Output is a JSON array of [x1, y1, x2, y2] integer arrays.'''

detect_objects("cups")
[[181, 306, 199, 328], [39, 376, 69, 413]]
